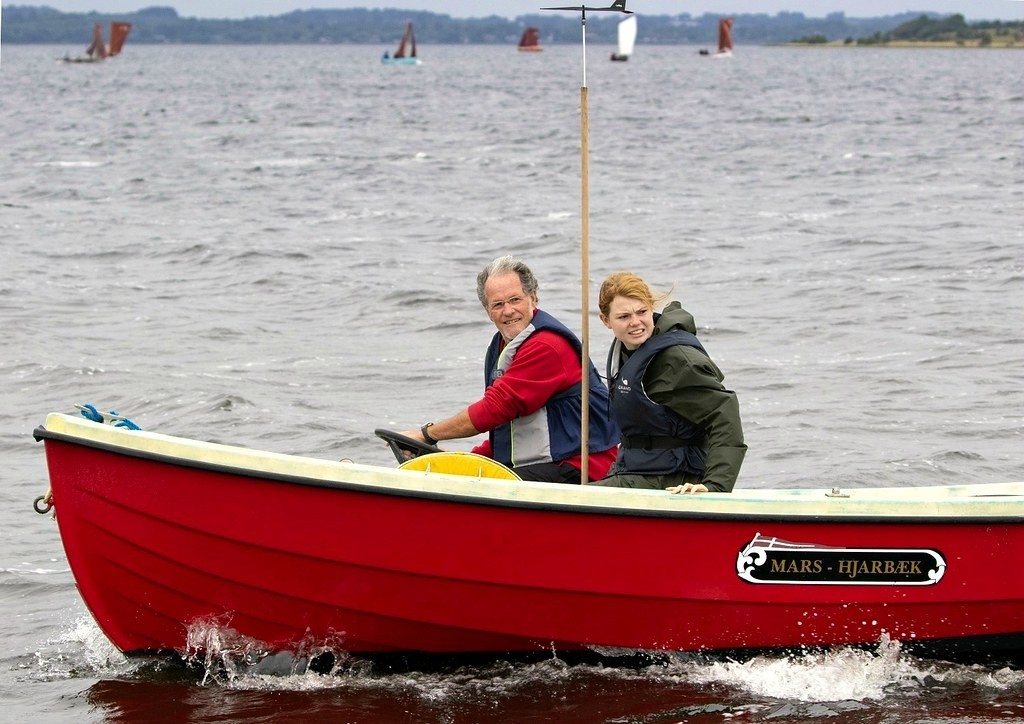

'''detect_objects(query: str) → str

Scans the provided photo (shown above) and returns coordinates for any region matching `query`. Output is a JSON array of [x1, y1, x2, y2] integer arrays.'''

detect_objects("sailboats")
[[380, 22, 419, 65], [518, 28, 543, 52], [712, 18, 733, 58], [58, 20, 131, 63], [609, 16, 638, 61]]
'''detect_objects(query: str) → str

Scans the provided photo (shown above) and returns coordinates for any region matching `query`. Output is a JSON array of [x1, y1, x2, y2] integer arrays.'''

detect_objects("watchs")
[[421, 422, 438, 445]]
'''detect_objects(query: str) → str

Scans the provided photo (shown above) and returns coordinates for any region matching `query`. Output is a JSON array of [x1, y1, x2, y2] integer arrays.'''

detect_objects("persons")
[[386, 255, 620, 485], [587, 272, 748, 494]]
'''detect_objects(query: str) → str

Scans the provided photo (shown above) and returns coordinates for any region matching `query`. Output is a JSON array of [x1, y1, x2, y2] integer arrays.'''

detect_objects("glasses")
[[486, 293, 532, 312]]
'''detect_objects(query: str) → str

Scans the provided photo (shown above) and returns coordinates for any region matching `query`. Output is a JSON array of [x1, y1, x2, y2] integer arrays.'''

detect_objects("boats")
[[35, 413, 1024, 663]]
